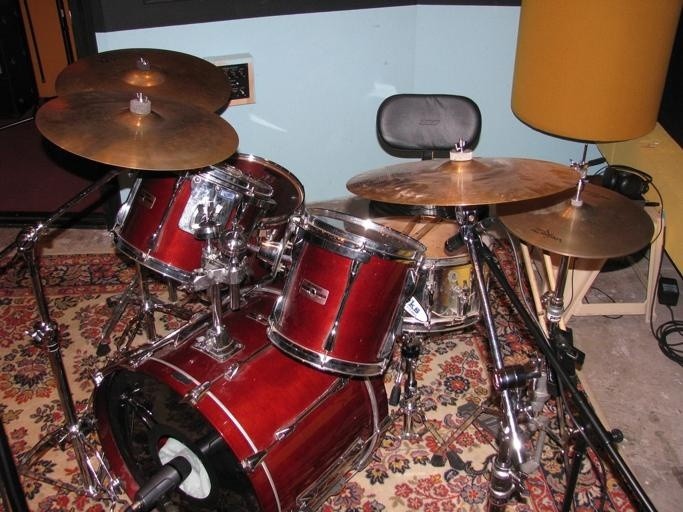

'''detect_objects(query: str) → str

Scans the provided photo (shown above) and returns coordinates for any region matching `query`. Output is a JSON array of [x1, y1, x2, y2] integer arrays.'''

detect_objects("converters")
[[658, 277, 679, 305]]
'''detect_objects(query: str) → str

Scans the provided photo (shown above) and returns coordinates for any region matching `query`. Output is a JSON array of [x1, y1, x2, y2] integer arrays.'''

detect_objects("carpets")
[[0, 210, 643, 511]]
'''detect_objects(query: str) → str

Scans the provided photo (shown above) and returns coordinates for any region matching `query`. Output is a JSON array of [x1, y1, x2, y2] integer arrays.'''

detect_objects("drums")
[[350, 210, 498, 334], [90, 287, 396, 512], [266, 207, 427, 377], [109, 162, 275, 290], [218, 155, 305, 292]]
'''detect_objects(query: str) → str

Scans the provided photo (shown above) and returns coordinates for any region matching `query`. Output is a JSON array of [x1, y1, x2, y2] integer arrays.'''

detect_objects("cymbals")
[[36, 96, 240, 172], [496, 183, 654, 258], [54, 47, 231, 114], [346, 155, 582, 207]]
